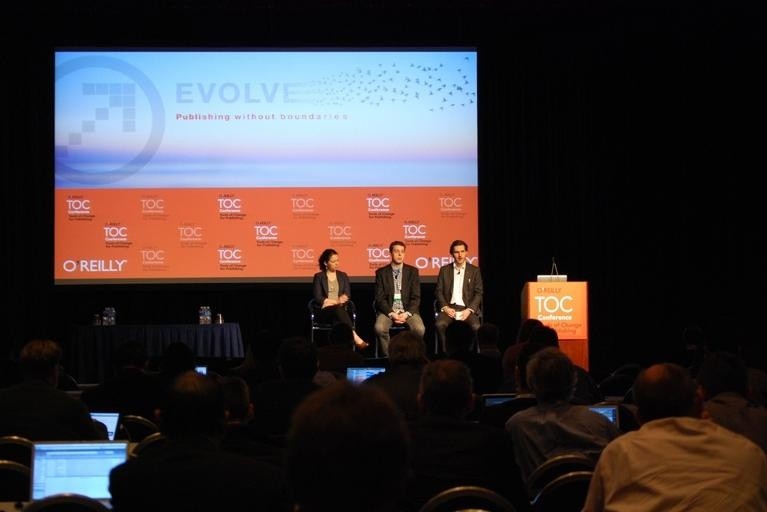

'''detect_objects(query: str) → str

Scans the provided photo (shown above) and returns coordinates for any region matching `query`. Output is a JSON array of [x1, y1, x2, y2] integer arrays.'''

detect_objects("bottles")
[[95, 307, 115, 326], [217, 313, 224, 325], [199, 307, 211, 325]]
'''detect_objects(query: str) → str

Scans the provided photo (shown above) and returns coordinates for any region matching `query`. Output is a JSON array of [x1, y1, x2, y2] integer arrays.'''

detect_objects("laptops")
[[481, 394, 516, 408], [346, 364, 386, 383], [29, 440, 129, 509], [195, 366, 207, 376], [588, 405, 619, 429], [90, 413, 119, 441]]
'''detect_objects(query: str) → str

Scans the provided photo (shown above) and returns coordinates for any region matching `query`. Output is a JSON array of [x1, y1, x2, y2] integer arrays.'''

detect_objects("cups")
[[454, 312, 463, 321]]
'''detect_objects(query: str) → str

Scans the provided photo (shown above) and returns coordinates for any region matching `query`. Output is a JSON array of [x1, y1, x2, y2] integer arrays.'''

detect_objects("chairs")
[[433, 295, 484, 354], [371, 297, 423, 359], [308, 297, 356, 353]]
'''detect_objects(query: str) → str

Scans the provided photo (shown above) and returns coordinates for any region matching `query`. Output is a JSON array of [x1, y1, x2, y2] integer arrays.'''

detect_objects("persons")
[[372, 241, 426, 358], [311, 247, 369, 351], [433, 240, 485, 355], [1, 318, 767, 511]]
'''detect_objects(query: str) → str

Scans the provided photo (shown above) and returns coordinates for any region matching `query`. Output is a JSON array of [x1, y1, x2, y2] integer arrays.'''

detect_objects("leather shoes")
[[354, 338, 370, 350]]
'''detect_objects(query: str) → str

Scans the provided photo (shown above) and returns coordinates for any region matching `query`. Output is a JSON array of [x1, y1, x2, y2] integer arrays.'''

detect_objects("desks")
[[84, 323, 245, 357]]
